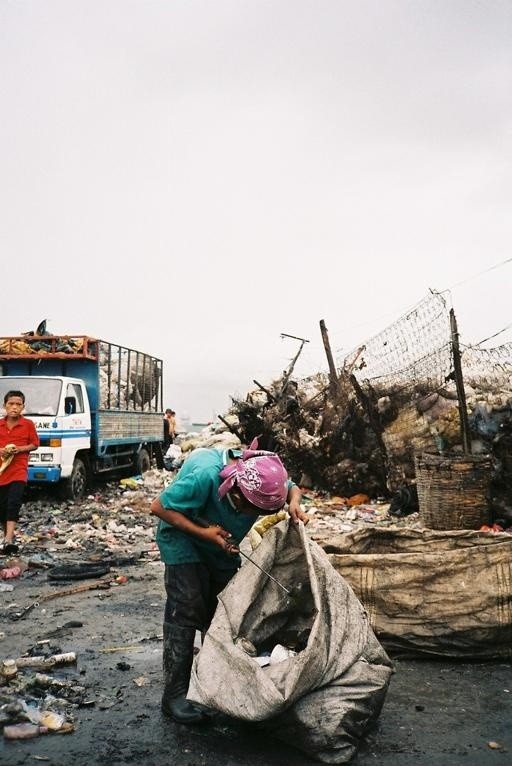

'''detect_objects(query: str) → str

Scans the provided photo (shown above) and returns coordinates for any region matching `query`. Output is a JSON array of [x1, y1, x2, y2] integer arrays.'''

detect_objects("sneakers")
[[0, 532, 19, 553]]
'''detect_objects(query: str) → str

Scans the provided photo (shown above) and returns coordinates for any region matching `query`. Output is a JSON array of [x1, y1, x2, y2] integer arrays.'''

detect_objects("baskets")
[[412, 443, 501, 533]]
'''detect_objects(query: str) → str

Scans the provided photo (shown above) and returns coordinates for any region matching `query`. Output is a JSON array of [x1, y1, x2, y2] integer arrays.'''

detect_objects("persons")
[[0, 390, 40, 553], [165, 408, 175, 444], [163, 418, 172, 454], [147, 436, 309, 724], [171, 411, 176, 417]]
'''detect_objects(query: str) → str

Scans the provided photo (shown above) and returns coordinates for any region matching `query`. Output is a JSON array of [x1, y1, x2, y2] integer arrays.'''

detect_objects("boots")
[[159, 621, 203, 725]]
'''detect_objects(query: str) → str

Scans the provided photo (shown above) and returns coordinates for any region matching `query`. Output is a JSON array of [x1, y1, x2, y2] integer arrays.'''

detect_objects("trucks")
[[0, 335, 164, 501]]
